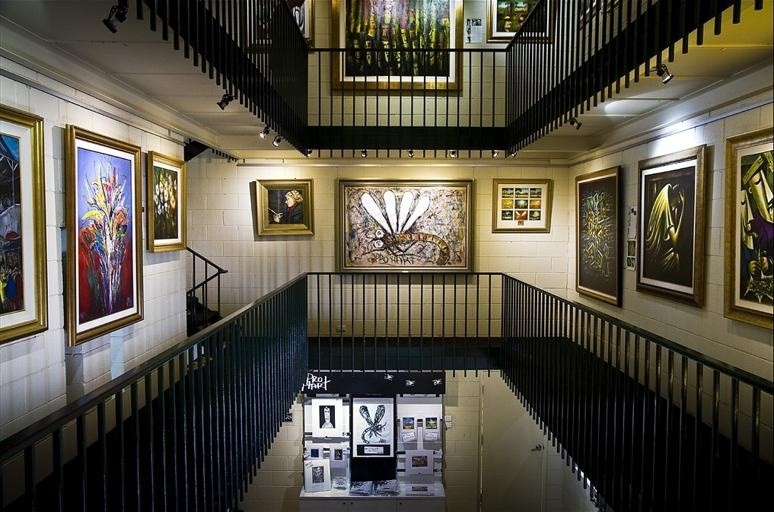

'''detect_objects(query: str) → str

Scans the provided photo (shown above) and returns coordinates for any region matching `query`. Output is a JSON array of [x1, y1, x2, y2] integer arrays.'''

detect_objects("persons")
[[321, 406, 333, 428], [153, 171, 177, 238], [644, 184, 685, 282], [0, 193, 19, 214], [465, 18, 472, 43], [313, 467, 323, 484], [273, 191, 303, 225], [346, 1, 449, 75], [0, 264, 25, 311]]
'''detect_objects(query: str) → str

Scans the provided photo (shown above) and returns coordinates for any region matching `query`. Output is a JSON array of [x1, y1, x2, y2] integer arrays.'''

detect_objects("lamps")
[[653, 65, 673, 85], [102, 6, 129, 35]]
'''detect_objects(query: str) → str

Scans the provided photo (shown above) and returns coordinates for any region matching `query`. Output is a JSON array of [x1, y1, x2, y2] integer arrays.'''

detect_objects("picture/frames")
[[147, 150, 187, 254], [722, 121, 774, 329], [255, 178, 316, 237], [0, 101, 51, 347], [635, 141, 708, 309], [491, 178, 554, 233], [573, 164, 624, 308], [64, 122, 145, 348], [334, 177, 475, 276], [299, 394, 446, 499]]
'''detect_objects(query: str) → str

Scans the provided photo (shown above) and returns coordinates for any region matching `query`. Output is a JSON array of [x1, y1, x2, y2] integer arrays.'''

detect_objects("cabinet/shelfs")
[[297, 499, 397, 512], [398, 500, 445, 512]]
